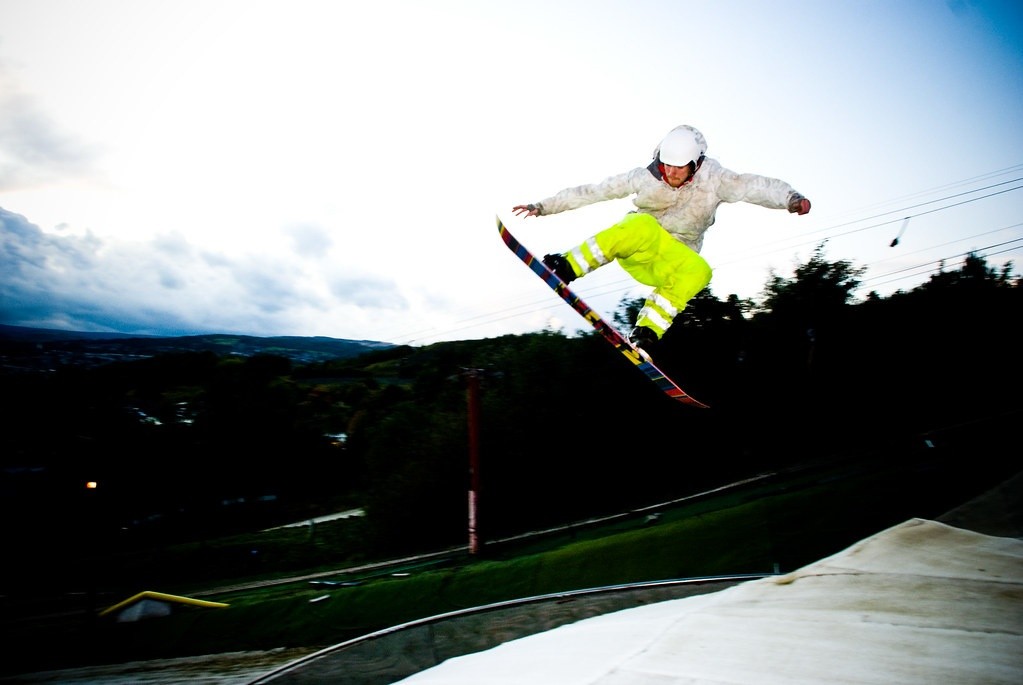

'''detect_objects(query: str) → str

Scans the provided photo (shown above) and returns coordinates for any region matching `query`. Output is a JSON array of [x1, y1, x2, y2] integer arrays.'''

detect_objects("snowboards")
[[493, 211, 713, 410]]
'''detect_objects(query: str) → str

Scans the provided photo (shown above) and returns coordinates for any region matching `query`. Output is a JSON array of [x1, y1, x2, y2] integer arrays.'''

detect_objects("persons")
[[512, 125, 811, 363]]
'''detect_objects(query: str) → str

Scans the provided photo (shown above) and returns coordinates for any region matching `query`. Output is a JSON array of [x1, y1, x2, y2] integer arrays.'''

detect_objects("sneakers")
[[542, 252, 578, 286], [627, 326, 659, 364]]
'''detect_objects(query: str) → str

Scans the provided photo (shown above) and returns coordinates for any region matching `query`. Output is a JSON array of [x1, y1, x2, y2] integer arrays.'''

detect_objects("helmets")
[[659, 129, 701, 170]]
